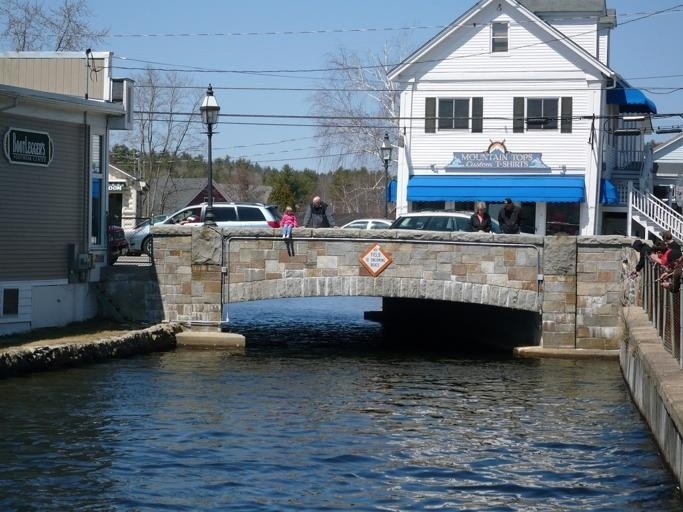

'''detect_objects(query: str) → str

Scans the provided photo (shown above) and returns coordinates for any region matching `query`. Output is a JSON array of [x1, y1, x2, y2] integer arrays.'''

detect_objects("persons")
[[279, 206, 297, 239], [303, 196, 338, 229], [498, 198, 522, 234], [470, 201, 492, 233], [631, 231, 683, 293]]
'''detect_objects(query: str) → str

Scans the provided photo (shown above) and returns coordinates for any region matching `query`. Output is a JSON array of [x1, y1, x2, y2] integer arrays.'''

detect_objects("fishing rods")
[[579, 271, 665, 318]]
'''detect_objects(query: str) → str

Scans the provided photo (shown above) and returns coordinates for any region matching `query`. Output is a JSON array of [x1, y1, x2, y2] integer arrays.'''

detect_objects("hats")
[[667, 250, 682, 262], [504, 199, 512, 204]]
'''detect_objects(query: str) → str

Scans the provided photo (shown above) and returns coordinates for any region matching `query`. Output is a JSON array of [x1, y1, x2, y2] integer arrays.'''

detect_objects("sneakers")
[[282, 234, 290, 239]]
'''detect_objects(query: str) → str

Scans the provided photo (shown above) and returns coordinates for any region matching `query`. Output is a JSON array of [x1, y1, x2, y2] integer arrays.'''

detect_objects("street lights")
[[199, 84, 220, 227], [381, 131, 393, 219]]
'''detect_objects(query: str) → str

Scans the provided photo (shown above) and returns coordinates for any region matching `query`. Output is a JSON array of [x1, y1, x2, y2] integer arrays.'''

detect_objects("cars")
[[110, 202, 502, 257]]
